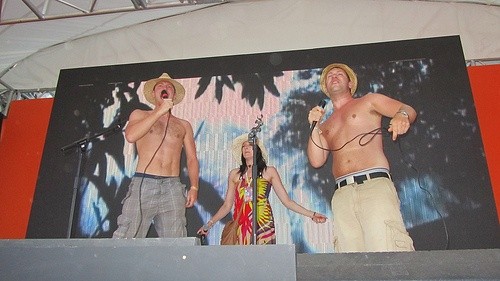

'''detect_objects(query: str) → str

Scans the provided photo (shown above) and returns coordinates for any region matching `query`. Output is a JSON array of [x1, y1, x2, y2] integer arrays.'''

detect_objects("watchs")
[[399, 110, 409, 118]]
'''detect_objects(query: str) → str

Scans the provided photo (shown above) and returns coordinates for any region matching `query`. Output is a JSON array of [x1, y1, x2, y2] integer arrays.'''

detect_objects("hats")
[[319, 63, 357, 98], [232, 133, 268, 165], [143, 72, 185, 105]]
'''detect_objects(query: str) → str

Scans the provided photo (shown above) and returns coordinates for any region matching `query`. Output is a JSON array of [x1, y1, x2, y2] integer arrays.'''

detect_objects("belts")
[[334, 171, 392, 190]]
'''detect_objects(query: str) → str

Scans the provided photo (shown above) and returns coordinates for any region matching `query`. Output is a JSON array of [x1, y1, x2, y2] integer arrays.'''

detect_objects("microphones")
[[310, 99, 326, 131], [161, 90, 170, 112]]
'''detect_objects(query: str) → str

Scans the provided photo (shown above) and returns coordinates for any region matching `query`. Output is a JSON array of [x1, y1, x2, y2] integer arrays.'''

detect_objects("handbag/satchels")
[[221, 219, 239, 245]]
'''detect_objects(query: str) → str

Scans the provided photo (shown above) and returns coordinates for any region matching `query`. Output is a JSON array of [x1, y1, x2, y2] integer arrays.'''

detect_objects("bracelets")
[[312, 212, 315, 220], [191, 186, 197, 191], [210, 219, 214, 224]]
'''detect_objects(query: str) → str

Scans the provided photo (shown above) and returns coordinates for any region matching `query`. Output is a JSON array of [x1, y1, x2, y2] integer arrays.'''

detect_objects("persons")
[[307, 63, 417, 252], [196, 133, 329, 245], [112, 73, 198, 239]]
